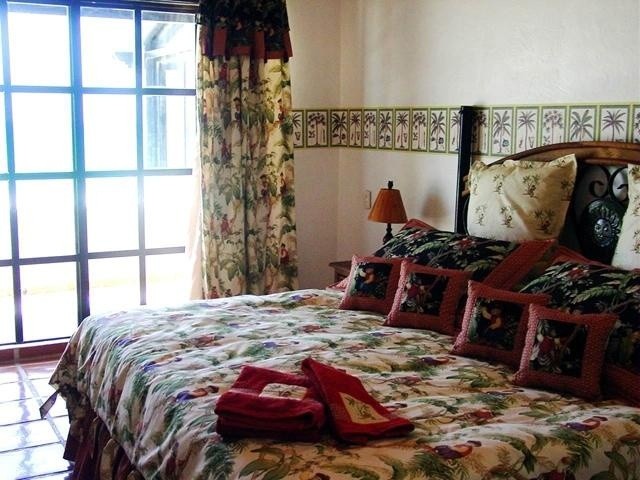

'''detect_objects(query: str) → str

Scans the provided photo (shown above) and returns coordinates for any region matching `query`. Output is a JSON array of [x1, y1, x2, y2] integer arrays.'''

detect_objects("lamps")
[[368, 181, 408, 245]]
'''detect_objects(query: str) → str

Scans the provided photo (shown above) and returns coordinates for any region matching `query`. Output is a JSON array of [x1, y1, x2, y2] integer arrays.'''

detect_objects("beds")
[[40, 140, 640, 480]]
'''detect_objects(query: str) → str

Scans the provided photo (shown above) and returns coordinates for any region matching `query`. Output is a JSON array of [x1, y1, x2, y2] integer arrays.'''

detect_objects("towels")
[[214, 366, 325, 443], [301, 358, 415, 446]]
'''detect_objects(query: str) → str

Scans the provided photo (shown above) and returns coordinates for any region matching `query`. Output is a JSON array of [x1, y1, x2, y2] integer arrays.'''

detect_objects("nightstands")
[[329, 260, 351, 283]]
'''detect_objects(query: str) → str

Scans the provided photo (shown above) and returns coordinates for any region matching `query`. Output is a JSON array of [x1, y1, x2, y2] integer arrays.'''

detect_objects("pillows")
[[445, 277, 555, 361], [508, 303, 620, 401], [511, 244, 639, 400], [341, 218, 557, 321], [379, 258, 469, 339], [340, 253, 411, 318], [460, 154, 578, 243], [610, 162, 640, 272]]
